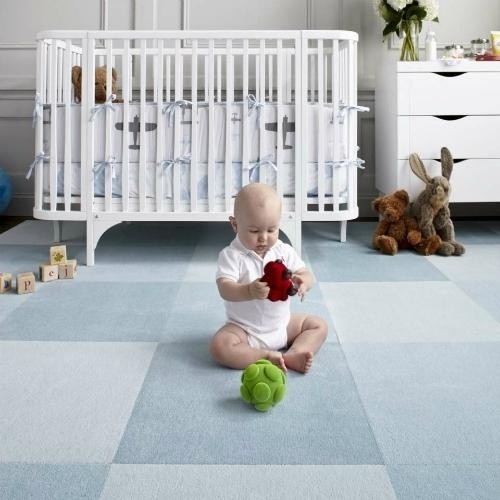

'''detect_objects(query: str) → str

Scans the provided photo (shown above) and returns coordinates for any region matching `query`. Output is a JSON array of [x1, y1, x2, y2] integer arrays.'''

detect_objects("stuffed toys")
[[70, 64, 128, 105], [406, 144, 466, 256], [368, 189, 443, 257], [236, 356, 288, 414], [259, 257, 298, 303]]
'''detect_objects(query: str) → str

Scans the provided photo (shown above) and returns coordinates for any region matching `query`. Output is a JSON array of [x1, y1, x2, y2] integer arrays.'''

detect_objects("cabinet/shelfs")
[[374, 59, 500, 202]]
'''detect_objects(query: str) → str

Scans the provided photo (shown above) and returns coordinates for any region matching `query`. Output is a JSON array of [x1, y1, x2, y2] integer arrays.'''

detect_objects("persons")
[[209, 181, 329, 377]]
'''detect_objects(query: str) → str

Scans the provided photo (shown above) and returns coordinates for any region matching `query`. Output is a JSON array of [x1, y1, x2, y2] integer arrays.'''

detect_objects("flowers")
[[374, 0, 439, 62]]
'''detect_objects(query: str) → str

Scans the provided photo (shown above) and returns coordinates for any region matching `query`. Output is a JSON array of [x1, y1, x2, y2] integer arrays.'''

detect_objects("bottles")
[[444, 43, 465, 60], [425, 31, 437, 60], [471, 38, 489, 57]]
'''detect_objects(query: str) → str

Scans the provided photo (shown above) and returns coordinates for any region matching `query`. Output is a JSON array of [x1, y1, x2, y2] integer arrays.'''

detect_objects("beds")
[[32, 29, 360, 265]]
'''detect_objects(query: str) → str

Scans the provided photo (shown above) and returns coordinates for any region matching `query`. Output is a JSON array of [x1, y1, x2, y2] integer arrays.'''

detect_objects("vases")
[[398, 20, 419, 62]]
